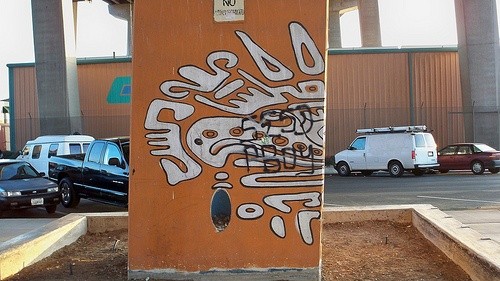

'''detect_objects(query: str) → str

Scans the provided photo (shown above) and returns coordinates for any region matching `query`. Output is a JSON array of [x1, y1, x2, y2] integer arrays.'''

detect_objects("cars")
[[0, 158, 61, 218], [437, 144, 499, 174]]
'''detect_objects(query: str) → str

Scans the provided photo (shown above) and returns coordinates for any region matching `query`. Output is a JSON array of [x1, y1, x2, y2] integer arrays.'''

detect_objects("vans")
[[333, 126, 440, 178], [15, 136, 95, 178]]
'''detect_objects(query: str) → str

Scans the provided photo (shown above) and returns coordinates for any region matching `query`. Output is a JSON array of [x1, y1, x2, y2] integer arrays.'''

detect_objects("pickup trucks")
[[48, 136, 129, 206]]
